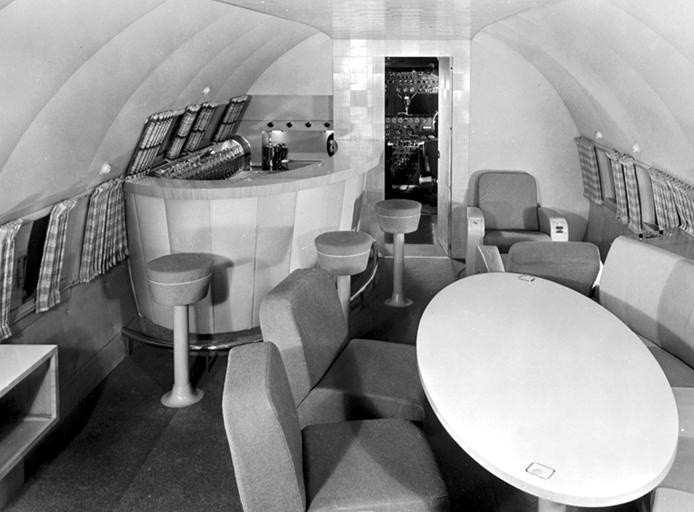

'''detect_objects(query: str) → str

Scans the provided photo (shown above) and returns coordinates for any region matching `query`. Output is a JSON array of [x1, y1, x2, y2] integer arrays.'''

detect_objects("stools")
[[314, 230, 373, 339], [145, 252, 215, 411], [376, 199, 422, 309]]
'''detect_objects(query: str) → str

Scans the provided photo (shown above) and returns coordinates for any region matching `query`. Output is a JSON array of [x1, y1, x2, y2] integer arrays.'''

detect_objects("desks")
[[415, 273, 679, 512], [1, 344, 61, 508]]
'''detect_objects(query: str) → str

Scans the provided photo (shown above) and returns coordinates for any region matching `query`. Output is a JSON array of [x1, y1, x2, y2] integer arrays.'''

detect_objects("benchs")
[[600, 234, 694, 512]]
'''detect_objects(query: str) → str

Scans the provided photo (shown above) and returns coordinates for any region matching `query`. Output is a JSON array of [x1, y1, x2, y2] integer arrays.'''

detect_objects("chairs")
[[258, 266, 429, 423], [475, 241, 605, 298], [220, 340, 449, 511], [452, 171, 570, 260]]
